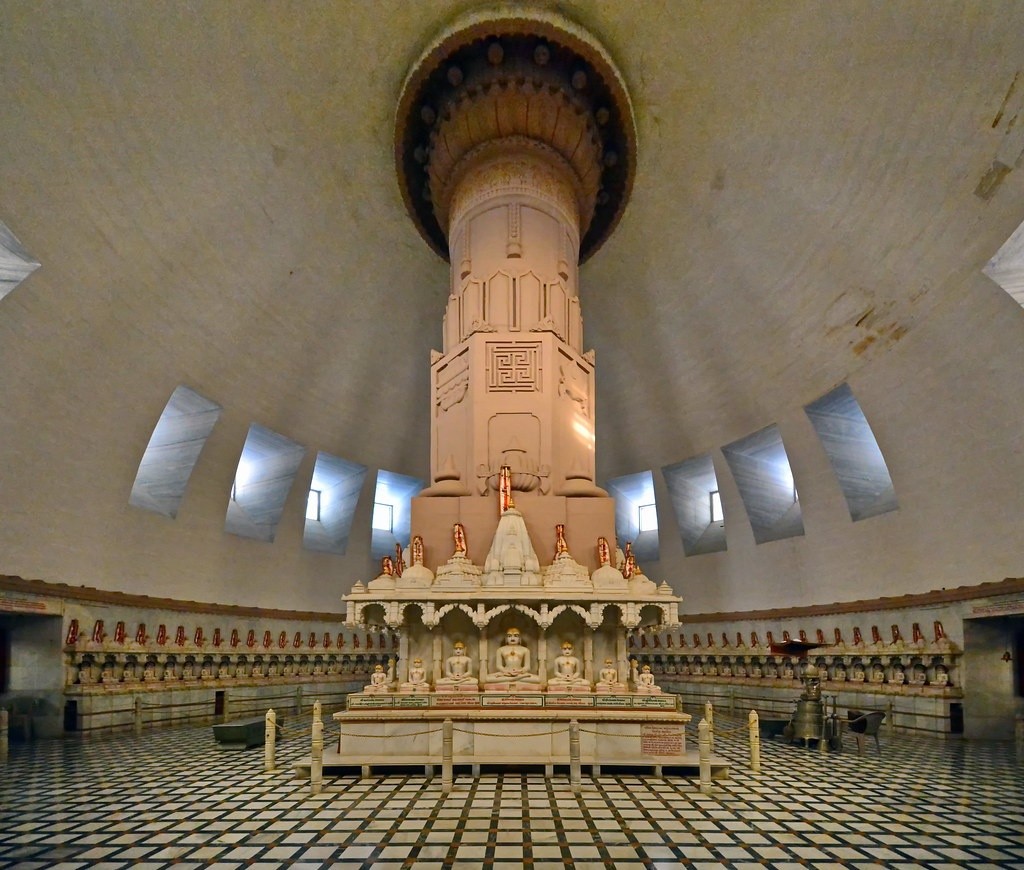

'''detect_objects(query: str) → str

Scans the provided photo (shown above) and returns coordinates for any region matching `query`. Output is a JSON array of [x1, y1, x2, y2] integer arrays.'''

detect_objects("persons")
[[363, 627, 662, 690], [667, 662, 949, 687], [79, 661, 362, 683]]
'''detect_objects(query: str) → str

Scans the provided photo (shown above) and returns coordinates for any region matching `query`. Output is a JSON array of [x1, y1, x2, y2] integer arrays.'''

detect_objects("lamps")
[[1001, 635, 1014, 661]]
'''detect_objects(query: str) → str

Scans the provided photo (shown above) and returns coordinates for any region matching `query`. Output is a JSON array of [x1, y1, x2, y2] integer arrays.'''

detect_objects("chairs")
[[840, 712, 885, 757]]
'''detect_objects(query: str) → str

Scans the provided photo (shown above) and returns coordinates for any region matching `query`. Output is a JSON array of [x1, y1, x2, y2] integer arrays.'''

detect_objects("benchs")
[[759, 718, 790, 739], [212, 716, 285, 752]]
